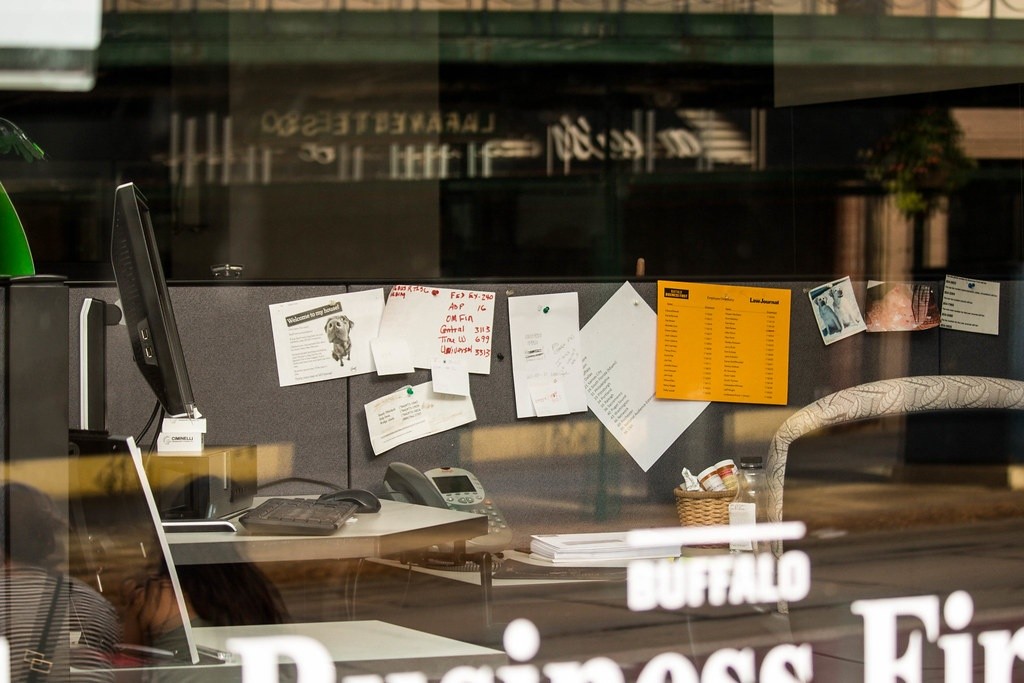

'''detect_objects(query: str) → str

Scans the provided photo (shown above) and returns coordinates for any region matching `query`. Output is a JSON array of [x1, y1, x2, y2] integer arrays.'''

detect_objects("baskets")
[[673, 486, 769, 549]]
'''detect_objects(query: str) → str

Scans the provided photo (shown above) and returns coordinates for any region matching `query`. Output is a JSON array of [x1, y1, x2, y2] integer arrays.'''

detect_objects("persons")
[[0, 476, 294, 683]]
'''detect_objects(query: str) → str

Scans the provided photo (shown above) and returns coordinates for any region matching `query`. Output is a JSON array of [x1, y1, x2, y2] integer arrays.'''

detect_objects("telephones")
[[380, 461, 513, 560]]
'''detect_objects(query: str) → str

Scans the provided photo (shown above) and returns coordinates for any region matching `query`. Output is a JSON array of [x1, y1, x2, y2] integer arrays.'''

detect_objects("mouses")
[[317, 489, 381, 514]]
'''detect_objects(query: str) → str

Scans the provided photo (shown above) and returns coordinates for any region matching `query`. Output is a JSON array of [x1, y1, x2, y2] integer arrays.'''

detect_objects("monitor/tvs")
[[66, 182, 197, 456]]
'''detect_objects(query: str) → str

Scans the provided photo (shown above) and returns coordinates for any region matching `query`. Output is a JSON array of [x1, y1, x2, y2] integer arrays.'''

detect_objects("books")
[[529, 531, 683, 563]]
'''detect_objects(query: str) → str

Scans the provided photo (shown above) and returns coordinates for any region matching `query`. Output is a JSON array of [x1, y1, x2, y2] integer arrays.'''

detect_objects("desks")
[[53, 494, 489, 628]]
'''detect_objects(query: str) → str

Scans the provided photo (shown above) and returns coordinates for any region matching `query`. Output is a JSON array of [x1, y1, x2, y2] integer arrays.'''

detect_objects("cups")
[[210, 263, 244, 286]]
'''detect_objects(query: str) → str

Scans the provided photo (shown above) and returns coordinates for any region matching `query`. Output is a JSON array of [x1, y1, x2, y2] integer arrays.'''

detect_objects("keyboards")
[[239, 498, 359, 536]]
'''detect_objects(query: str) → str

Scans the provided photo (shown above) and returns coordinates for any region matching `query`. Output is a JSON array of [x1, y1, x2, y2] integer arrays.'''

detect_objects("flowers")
[[864, 98, 976, 216]]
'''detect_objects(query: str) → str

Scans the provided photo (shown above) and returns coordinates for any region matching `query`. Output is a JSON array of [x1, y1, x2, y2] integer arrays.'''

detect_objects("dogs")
[[814, 287, 860, 336], [324, 316, 354, 367]]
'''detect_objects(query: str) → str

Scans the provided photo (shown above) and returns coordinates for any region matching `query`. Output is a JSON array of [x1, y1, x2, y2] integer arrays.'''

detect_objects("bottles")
[[714, 460, 739, 490], [728, 457, 777, 553], [697, 466, 727, 492]]
